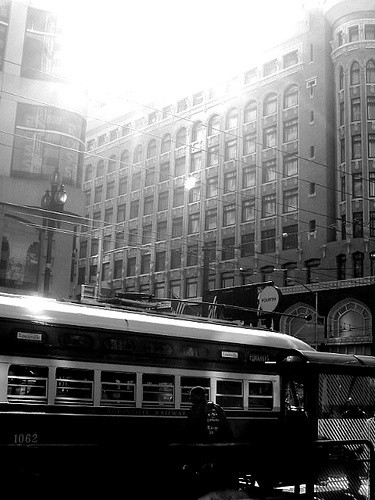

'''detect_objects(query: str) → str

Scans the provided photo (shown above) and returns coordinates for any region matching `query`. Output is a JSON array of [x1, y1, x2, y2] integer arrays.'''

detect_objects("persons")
[[184, 386, 234, 444]]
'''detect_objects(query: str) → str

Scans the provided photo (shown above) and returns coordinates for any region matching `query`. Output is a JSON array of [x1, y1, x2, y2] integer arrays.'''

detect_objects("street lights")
[[39, 164, 68, 297], [286, 278, 318, 351]]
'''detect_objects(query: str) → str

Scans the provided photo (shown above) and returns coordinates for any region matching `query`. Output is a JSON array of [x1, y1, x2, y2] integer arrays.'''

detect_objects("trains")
[[1, 292, 327, 498]]
